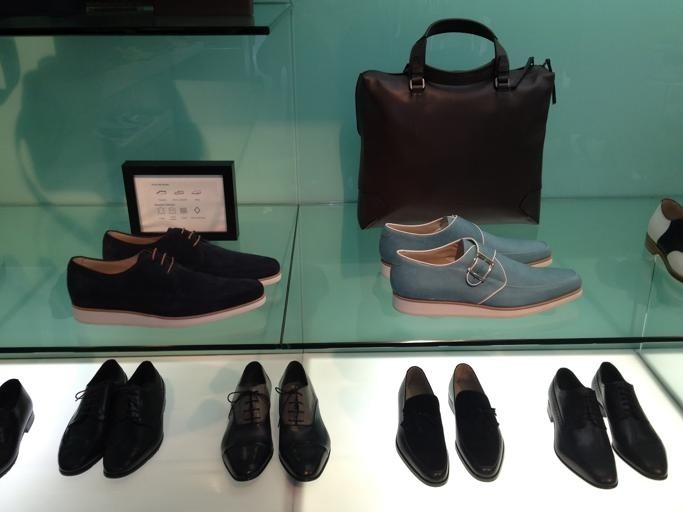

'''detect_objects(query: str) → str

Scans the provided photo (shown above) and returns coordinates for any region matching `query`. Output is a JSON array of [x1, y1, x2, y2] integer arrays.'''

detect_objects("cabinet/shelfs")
[[0, 2, 683, 512]]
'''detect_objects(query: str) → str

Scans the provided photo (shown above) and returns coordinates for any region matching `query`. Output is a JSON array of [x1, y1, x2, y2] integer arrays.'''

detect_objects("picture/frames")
[[121, 160, 240, 241]]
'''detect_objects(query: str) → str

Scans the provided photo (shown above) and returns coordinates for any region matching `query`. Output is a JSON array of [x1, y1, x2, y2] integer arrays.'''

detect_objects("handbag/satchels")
[[356, 18, 556, 228]]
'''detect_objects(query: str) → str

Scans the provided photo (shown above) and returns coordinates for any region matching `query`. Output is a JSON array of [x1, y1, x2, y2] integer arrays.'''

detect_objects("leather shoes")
[[1, 377, 35, 476], [547, 359, 667, 489], [276, 361, 331, 480], [447, 363, 504, 482], [645, 197, 683, 282], [220, 360, 274, 481], [67, 225, 281, 328], [378, 216, 582, 319], [396, 364, 450, 487], [58, 360, 166, 479]]
